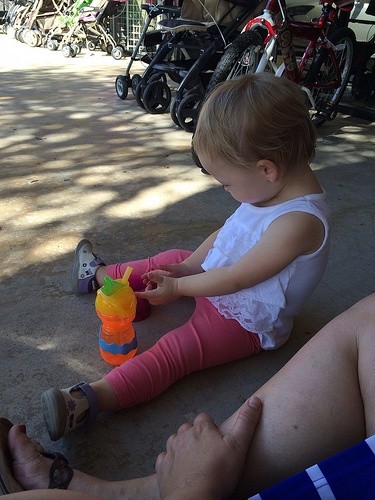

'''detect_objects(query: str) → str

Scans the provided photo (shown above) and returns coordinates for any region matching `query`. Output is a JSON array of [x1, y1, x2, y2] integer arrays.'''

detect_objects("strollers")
[[3, 0, 124, 59], [115, 0, 258, 134]]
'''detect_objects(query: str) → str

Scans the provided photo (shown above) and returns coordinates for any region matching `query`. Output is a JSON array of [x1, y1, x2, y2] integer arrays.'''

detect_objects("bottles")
[[95, 265, 136, 365]]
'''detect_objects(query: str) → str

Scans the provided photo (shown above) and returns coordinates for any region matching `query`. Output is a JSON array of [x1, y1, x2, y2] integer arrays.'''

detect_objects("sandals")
[[42, 381, 97, 441], [0, 418, 73, 493], [71, 240, 105, 296]]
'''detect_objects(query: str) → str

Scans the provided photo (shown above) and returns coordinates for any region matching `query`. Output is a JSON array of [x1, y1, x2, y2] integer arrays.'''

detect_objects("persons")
[[40, 72, 329, 441], [0, 293, 375, 500]]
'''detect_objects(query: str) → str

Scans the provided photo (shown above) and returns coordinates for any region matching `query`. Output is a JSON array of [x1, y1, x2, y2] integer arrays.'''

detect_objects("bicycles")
[[192, 0, 356, 173]]
